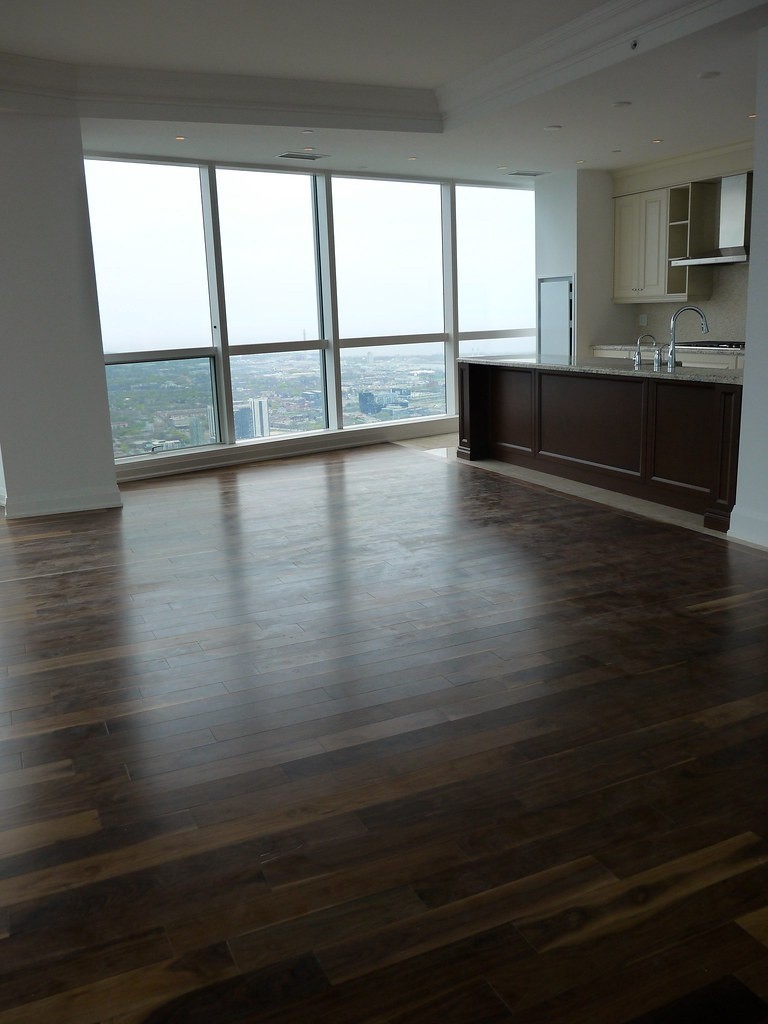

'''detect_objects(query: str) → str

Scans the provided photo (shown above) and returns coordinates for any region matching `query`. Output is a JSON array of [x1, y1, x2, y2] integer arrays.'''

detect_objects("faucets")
[[622, 334, 657, 373], [667, 306, 710, 373], [652, 343, 669, 374]]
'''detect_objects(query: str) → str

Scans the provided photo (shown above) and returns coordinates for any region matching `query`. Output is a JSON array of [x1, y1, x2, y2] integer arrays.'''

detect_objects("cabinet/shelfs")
[[611, 183, 717, 305]]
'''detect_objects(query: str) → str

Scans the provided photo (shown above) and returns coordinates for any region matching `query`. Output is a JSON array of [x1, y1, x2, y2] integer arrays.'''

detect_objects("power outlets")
[[638, 313, 648, 327]]
